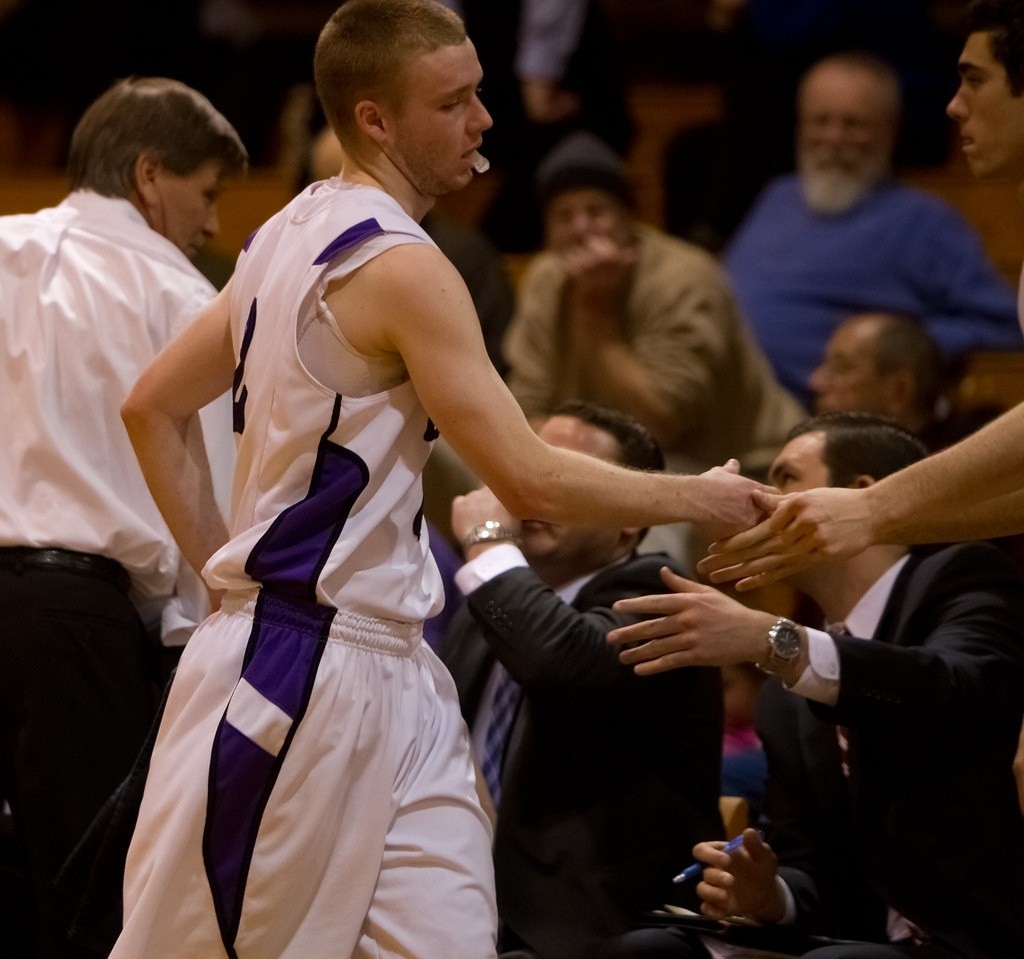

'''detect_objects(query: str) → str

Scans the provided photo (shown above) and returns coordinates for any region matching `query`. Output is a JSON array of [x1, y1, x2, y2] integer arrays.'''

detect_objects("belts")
[[0, 547, 133, 596]]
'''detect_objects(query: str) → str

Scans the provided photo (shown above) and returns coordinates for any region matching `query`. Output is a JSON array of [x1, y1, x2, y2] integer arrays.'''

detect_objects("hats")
[[536, 133, 641, 218]]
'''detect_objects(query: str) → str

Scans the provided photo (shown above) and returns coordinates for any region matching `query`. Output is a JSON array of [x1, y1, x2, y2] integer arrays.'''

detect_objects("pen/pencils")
[[672, 829, 763, 883]]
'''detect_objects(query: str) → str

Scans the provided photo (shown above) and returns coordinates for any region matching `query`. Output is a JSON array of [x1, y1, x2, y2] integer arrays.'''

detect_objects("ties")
[[834, 626, 935, 955], [481, 586, 555, 812]]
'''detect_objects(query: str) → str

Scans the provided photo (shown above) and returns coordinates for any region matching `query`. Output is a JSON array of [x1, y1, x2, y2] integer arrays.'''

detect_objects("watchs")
[[462, 520, 514, 554], [755, 617, 801, 676]]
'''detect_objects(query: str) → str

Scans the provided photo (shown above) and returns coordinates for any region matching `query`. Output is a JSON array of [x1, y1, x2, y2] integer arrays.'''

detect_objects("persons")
[[436, 400, 725, 959], [108, 0, 782, 959], [605, 410, 1024, 959], [0, 76, 249, 959], [277, 0, 1024, 959]]
